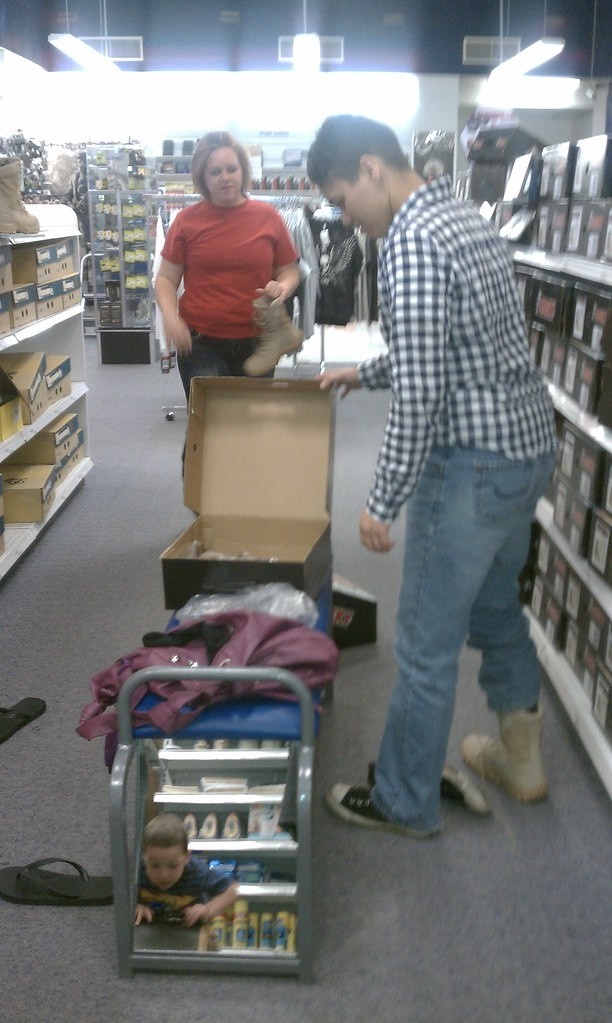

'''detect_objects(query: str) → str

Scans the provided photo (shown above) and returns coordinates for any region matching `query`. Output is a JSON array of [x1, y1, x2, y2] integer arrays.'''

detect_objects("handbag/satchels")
[[74, 609, 339, 741]]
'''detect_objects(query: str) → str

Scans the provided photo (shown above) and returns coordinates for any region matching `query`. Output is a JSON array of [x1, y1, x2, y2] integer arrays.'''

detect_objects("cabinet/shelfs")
[[86, 135, 196, 366], [154, 753, 295, 957], [505, 243, 611, 797], [1, 205, 97, 578]]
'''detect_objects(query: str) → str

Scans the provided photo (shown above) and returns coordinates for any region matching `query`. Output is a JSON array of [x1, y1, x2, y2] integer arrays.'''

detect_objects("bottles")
[[198, 900, 296, 953]]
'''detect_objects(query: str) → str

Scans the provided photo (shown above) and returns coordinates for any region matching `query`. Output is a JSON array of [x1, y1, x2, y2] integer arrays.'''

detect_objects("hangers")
[[272, 195, 303, 213]]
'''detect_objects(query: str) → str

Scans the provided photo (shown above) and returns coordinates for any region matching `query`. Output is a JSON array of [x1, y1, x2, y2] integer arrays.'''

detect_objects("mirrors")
[[136, 749, 298, 960]]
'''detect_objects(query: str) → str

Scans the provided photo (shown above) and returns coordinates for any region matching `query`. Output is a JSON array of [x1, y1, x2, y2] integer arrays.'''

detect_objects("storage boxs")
[[467, 127, 612, 742], [0, 239, 81, 333], [115, 376, 378, 753], [0, 351, 84, 553]]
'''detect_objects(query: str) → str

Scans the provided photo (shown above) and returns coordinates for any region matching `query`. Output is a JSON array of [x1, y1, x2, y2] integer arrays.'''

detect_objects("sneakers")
[[324, 782, 444, 837], [368, 758, 491, 816]]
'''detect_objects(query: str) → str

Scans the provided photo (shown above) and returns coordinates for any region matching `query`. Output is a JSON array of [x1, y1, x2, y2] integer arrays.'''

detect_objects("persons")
[[134, 813, 239, 931], [152, 131, 301, 517], [306, 116, 557, 836]]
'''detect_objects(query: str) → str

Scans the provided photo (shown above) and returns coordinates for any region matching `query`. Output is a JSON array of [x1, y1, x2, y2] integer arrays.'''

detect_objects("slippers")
[[0, 697, 47, 744], [0, 857, 113, 906]]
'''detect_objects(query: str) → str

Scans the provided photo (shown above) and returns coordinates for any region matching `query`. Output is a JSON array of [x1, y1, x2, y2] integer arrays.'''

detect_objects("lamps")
[[293, 0, 321, 73], [488, 0, 566, 76], [48, 0, 120, 71]]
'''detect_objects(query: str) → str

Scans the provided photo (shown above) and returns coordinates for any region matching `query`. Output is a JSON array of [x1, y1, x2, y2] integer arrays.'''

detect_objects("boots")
[[0, 157, 39, 234], [461, 702, 549, 801], [241, 295, 304, 377]]
